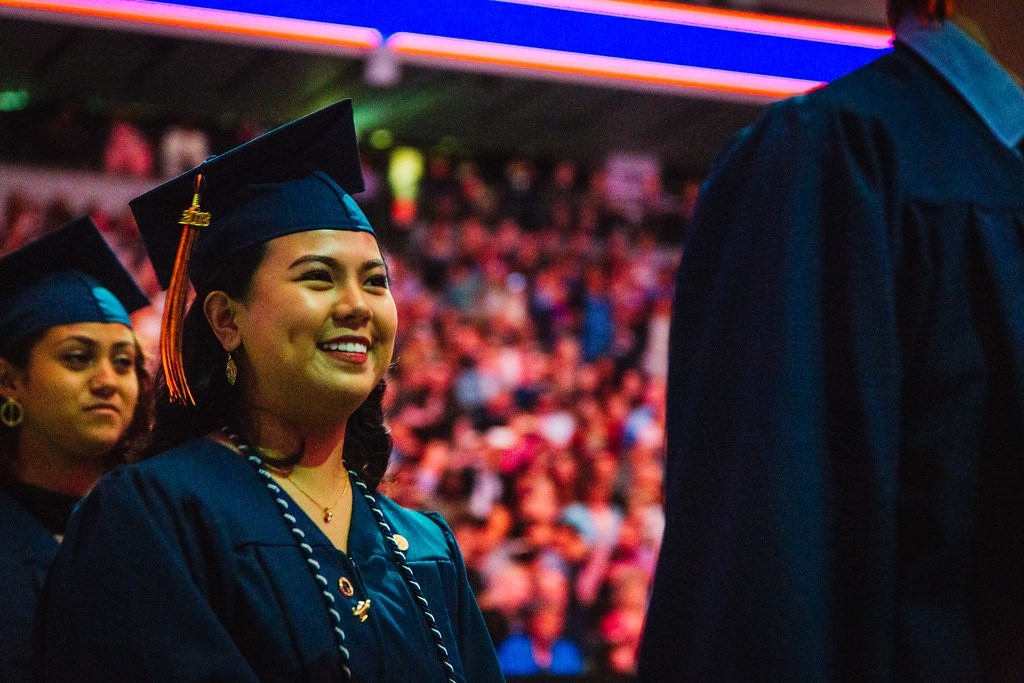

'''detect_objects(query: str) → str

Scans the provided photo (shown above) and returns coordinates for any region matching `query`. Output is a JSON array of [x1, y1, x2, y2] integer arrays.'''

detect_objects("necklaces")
[[284, 471, 349, 523]]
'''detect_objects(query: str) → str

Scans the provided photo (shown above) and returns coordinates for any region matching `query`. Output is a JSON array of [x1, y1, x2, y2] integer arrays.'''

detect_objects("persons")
[[0, 217, 152, 683], [0, 105, 266, 397], [32, 94, 505, 683], [345, 130, 708, 683], [634, 0, 1024, 683]]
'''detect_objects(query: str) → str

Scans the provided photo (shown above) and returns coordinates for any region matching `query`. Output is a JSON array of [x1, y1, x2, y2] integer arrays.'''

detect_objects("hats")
[[129, 98, 376, 408], [0, 216, 156, 432]]
